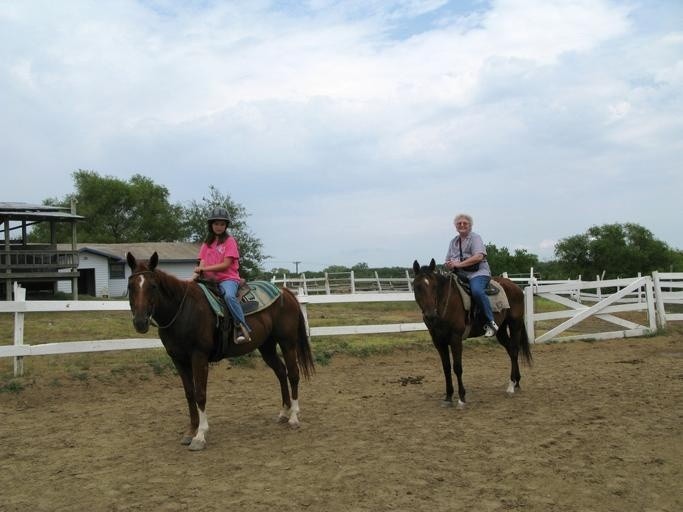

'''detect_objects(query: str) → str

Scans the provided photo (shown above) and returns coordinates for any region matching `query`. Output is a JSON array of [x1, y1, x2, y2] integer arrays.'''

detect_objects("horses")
[[413, 259, 533, 410], [126, 251, 316, 450]]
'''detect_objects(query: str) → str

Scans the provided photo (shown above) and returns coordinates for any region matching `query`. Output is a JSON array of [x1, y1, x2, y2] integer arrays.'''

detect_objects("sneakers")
[[236, 329, 251, 343], [484, 321, 498, 337]]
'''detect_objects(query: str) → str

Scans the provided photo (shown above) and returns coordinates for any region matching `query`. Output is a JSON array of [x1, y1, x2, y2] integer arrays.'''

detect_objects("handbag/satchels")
[[461, 257, 478, 271]]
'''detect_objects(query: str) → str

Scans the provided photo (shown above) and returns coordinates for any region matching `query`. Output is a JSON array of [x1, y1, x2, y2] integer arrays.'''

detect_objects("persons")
[[192, 205, 253, 344], [445, 213, 500, 338]]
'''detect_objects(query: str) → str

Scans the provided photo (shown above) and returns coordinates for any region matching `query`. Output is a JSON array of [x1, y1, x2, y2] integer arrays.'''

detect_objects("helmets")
[[207, 206, 230, 223]]
